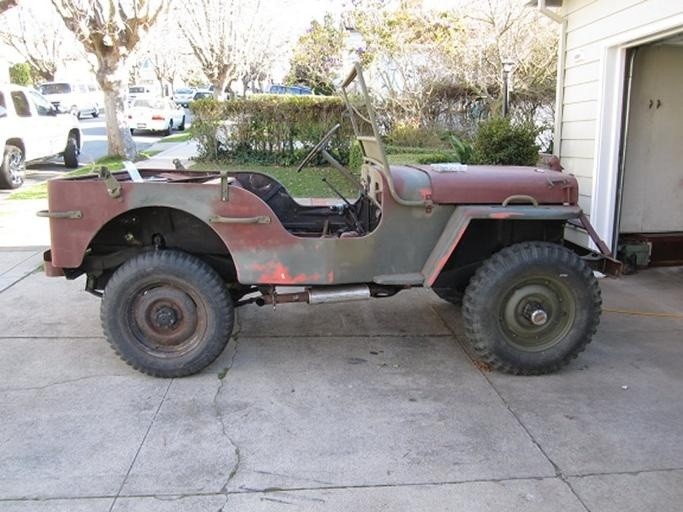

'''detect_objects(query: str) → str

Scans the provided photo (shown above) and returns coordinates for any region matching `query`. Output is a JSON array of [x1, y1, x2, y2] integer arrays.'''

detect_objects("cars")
[[128, 82, 156, 103], [38, 80, 101, 120], [124, 96, 186, 138], [172, 83, 235, 108], [0, 78, 85, 192]]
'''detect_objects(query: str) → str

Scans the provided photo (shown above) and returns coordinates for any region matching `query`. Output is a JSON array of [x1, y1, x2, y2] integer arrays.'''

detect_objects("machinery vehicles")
[[34, 56, 624, 379]]
